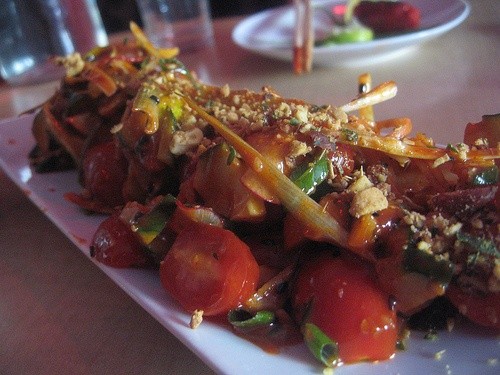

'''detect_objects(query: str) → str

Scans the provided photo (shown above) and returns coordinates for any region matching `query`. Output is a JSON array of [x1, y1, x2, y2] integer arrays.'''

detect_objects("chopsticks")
[[292, 0, 314, 76]]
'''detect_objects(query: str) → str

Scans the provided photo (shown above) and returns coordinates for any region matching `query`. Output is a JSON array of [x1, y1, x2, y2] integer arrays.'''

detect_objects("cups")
[[136, 0, 216, 58], [0, 0, 110, 87]]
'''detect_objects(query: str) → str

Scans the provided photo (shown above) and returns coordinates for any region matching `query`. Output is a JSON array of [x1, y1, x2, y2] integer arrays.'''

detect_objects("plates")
[[1, 110, 500, 375], [230, 0, 472, 71]]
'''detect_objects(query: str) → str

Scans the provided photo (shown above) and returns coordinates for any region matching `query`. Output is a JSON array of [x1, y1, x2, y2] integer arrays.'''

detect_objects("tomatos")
[[27, 40, 500, 363]]
